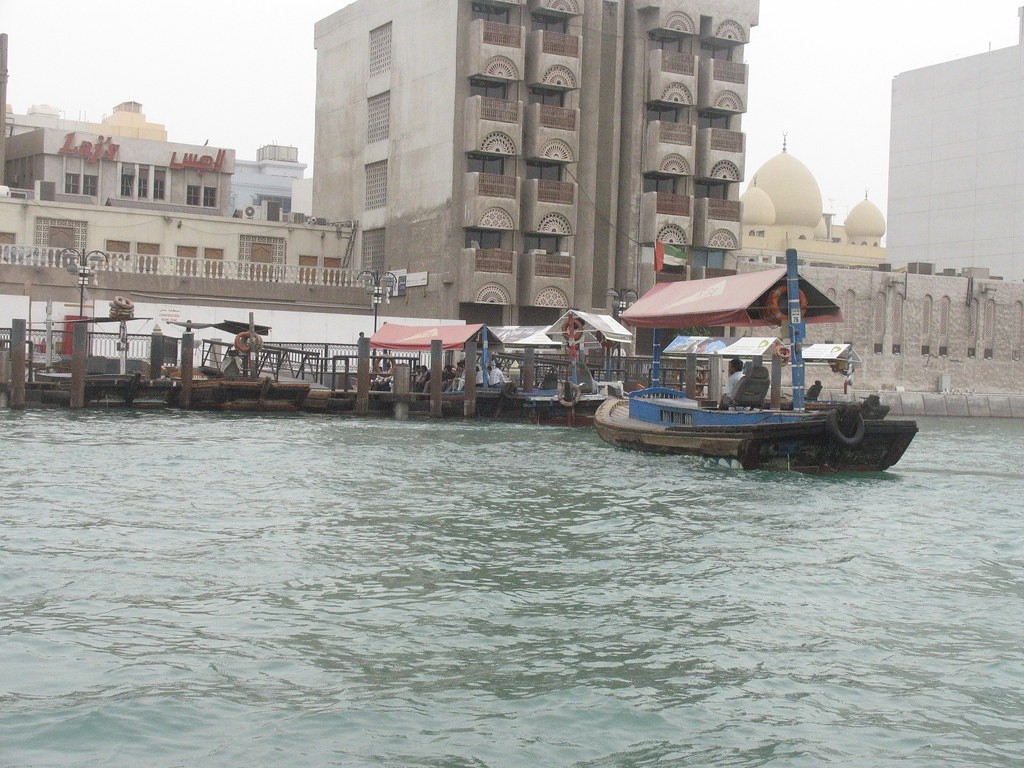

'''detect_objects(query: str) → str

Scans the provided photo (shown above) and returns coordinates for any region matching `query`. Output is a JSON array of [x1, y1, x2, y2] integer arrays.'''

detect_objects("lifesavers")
[[109, 296, 135, 321], [767, 285, 807, 324], [235, 332, 263, 353], [374, 355, 397, 376], [563, 321, 583, 341], [834, 356, 855, 377], [824, 401, 869, 447], [596, 331, 616, 346], [771, 345, 792, 365]]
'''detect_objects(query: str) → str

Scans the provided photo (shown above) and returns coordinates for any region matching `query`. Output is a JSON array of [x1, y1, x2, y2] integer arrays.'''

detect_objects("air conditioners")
[[243, 205, 261, 219]]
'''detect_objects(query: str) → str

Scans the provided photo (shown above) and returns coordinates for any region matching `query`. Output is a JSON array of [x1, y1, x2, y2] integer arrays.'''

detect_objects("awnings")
[[487, 325, 574, 389], [619, 265, 862, 410], [545, 308, 633, 384], [368, 321, 505, 392]]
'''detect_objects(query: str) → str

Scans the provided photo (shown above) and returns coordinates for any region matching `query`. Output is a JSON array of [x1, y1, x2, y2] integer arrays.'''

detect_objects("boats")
[[1, 319, 608, 426], [599, 250, 920, 474]]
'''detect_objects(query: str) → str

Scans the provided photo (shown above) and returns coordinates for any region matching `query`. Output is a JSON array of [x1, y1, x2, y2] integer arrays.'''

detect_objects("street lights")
[[56, 248, 111, 314], [351, 269, 400, 371]]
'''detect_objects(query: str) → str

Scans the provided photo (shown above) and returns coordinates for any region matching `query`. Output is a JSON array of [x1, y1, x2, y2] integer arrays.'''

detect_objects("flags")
[[653, 237, 688, 273]]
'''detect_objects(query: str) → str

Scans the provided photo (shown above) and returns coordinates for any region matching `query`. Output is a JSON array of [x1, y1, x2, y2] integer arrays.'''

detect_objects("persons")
[[371, 359, 510, 394], [718, 358, 746, 409]]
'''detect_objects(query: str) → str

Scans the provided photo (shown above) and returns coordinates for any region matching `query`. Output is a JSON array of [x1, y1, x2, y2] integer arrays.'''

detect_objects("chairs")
[[732, 360, 771, 407], [805, 381, 822, 403]]
